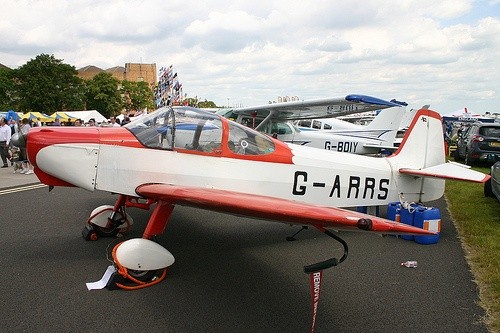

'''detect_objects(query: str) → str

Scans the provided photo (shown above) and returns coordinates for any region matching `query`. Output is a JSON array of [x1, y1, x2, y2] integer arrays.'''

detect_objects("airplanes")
[[11, 93, 492, 292]]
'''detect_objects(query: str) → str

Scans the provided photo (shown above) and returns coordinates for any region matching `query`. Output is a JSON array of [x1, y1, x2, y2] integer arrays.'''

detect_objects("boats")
[[443, 108, 495, 123]]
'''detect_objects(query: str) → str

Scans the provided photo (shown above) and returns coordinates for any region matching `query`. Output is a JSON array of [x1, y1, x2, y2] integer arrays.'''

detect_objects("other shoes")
[[10, 158, 15, 166], [1, 164, 8, 168], [20, 168, 29, 174], [18, 169, 22, 173], [13, 170, 16, 173], [25, 170, 34, 175]]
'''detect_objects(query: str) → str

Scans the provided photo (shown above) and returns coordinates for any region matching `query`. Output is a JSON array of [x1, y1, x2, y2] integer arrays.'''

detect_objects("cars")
[[484, 153, 500, 200]]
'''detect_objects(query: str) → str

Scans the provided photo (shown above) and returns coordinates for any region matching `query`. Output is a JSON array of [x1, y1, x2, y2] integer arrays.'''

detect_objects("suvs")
[[454, 121, 500, 166]]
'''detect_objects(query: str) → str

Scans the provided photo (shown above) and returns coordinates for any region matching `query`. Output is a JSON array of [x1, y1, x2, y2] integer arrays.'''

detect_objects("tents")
[[20, 110, 110, 123]]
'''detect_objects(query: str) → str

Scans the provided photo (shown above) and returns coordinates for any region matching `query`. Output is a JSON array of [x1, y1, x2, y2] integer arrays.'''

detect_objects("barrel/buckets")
[[387, 202, 442, 245]]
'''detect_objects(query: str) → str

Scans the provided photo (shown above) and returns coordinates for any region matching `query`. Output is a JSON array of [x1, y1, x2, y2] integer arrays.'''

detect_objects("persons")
[[74, 119, 85, 127], [18, 120, 23, 132], [450, 129, 463, 161], [62, 121, 66, 126], [108, 116, 121, 127], [228, 140, 235, 153], [18, 118, 33, 175], [9, 146, 24, 173], [65, 118, 73, 125], [0, 117, 14, 168], [5, 120, 11, 126], [271, 129, 278, 139], [121, 111, 131, 126], [81, 120, 84, 124], [445, 125, 453, 142], [86, 118, 96, 127], [33, 117, 42, 127], [9, 120, 16, 135], [51, 118, 60, 125]]
